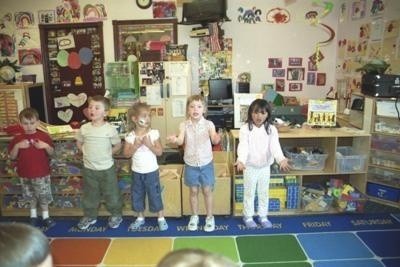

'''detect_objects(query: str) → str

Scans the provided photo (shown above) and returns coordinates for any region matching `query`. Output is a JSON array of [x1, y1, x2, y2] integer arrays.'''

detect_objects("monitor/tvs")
[[181, 0, 224, 21], [209, 78, 232, 104]]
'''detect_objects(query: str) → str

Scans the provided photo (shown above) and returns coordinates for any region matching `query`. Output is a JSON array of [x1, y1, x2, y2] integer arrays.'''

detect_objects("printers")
[[360, 74, 400, 97]]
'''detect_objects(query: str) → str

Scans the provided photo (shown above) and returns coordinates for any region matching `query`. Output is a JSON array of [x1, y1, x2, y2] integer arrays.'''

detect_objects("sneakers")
[[29, 218, 38, 227], [159, 219, 168, 231], [259, 218, 273, 230], [187, 215, 199, 231], [42, 218, 56, 231], [243, 216, 256, 228], [108, 215, 123, 228], [78, 217, 97, 229], [129, 218, 145, 230], [204, 216, 215, 232]]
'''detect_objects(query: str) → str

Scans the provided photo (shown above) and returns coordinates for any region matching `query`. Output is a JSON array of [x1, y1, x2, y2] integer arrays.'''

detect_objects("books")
[[47, 124, 75, 137]]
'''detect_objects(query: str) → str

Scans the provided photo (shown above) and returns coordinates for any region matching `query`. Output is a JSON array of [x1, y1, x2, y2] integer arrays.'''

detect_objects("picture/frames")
[[112, 18, 179, 62]]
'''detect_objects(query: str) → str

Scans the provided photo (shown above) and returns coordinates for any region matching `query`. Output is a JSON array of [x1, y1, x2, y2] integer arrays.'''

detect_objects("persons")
[[7, 107, 56, 229], [155, 247, 239, 266], [166, 93, 224, 232], [233, 98, 293, 229], [123, 101, 169, 232], [0, 222, 53, 267], [75, 94, 125, 229]]
